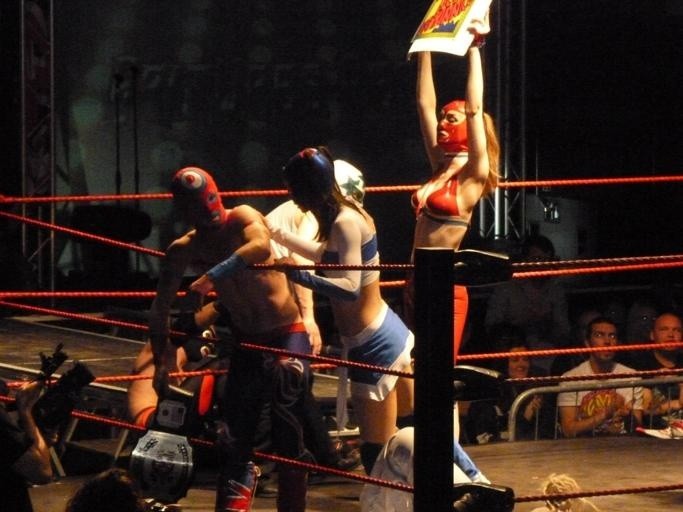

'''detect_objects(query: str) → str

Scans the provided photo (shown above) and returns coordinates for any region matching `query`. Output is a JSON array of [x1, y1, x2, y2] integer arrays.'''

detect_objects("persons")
[[463, 233, 681, 442], [0, 380, 58, 512], [65, 469, 143, 512], [267, 147, 493, 488], [159, 165, 312, 512], [127, 301, 272, 484], [264, 158, 367, 358], [402, 8, 499, 364]]
